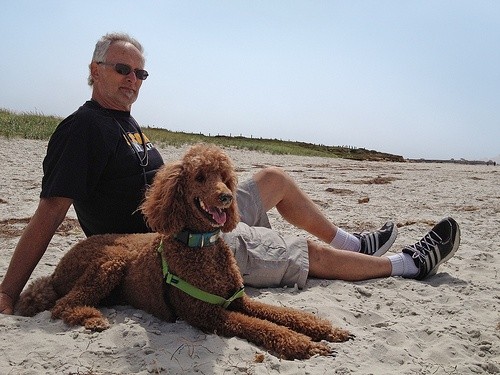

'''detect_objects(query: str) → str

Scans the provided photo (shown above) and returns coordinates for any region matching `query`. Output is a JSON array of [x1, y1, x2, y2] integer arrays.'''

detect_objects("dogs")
[[14, 141, 356, 360]]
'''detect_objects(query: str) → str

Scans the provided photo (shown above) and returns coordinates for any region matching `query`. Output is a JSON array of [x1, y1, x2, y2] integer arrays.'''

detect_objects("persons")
[[0, 32, 461, 316]]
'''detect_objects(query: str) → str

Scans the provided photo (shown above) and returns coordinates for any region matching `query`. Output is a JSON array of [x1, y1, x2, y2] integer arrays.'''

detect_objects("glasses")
[[96, 62, 149, 80]]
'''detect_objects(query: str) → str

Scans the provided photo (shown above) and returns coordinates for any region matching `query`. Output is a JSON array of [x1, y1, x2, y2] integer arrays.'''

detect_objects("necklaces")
[[91, 97, 149, 166]]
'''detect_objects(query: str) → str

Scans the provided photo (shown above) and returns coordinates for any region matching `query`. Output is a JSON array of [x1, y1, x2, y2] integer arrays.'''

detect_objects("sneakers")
[[402, 217, 461, 281], [353, 222, 398, 257]]
